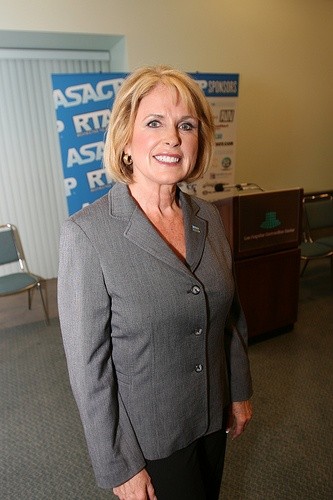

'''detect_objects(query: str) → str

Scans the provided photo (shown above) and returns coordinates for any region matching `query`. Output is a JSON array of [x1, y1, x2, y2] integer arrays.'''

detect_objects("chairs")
[[0, 224, 50, 326], [294, 191, 333, 276]]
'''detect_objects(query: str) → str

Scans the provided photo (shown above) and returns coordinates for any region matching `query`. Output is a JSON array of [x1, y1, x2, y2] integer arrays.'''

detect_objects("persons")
[[58, 67, 254, 500]]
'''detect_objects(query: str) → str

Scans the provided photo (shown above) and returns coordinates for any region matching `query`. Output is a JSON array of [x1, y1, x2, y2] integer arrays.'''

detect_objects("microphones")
[[214, 183, 249, 192]]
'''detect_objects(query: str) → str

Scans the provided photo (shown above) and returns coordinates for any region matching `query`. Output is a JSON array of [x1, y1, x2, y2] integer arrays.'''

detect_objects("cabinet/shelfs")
[[212, 189, 304, 345]]
